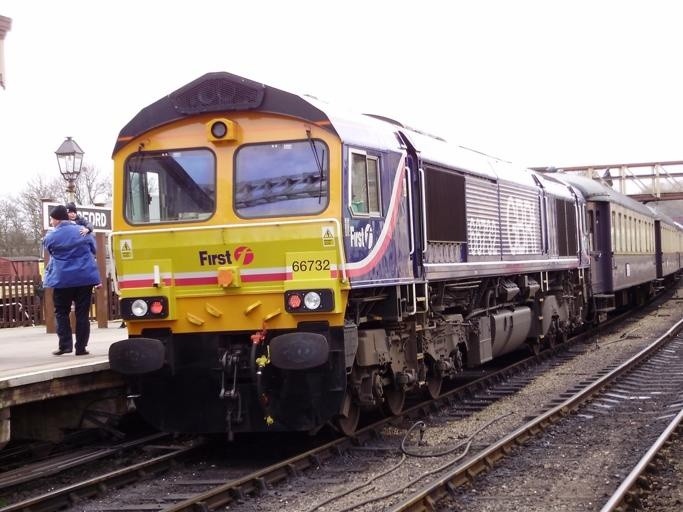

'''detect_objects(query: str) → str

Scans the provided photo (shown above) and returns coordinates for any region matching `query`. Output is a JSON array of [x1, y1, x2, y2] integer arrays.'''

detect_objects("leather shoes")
[[52, 345, 91, 355]]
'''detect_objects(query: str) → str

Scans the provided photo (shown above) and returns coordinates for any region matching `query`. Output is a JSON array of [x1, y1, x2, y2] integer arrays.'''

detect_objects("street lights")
[[55, 135, 84, 204]]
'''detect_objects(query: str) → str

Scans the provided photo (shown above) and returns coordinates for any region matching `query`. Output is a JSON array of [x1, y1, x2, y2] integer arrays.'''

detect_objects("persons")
[[43, 205, 101, 355], [65, 202, 94, 237]]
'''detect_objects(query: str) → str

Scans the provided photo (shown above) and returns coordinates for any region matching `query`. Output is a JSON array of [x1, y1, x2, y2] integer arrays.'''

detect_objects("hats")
[[50, 202, 77, 220]]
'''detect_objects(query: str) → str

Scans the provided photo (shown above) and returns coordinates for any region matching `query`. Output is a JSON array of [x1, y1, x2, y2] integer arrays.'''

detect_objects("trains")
[[106, 71, 683, 437]]
